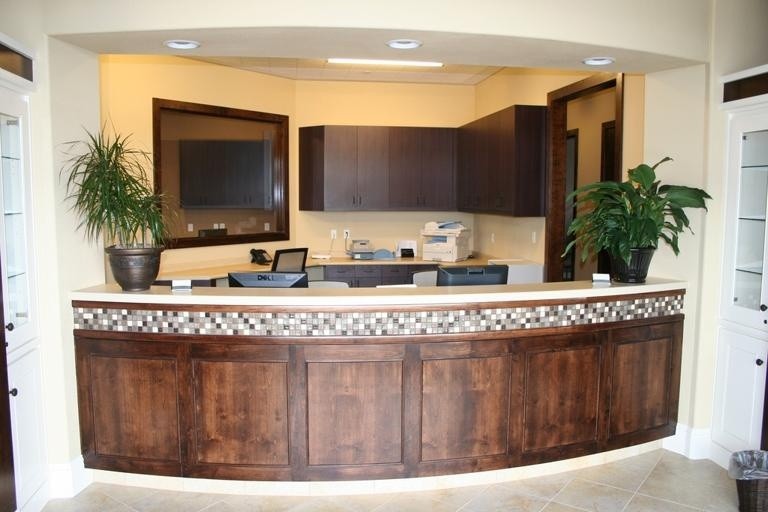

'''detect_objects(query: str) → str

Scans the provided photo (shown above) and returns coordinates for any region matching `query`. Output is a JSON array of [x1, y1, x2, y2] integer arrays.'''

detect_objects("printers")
[[422, 221, 471, 262]]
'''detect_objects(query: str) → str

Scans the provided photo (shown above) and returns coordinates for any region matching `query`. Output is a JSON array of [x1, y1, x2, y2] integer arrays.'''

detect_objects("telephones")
[[250, 249, 272, 264]]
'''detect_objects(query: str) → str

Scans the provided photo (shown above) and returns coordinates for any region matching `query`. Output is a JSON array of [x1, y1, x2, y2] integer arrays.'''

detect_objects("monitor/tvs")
[[437, 265, 508, 286], [228, 272, 308, 288], [199, 229, 227, 238]]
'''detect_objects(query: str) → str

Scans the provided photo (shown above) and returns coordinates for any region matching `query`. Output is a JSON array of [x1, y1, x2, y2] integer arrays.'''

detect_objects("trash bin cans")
[[732, 450, 768, 512]]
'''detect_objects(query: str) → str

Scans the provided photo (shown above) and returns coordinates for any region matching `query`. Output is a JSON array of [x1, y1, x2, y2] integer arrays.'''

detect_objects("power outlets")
[[344, 230, 349, 238]]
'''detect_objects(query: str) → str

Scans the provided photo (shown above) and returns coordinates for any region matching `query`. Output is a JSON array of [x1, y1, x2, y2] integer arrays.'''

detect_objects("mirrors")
[[152, 98, 289, 250]]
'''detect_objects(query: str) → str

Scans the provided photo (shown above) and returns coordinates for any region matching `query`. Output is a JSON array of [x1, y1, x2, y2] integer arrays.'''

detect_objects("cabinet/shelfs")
[[0, 85, 50, 512], [457, 104, 548, 217], [389, 126, 457, 211], [325, 264, 438, 287], [179, 139, 274, 209], [299, 125, 389, 211], [710, 108, 768, 456]]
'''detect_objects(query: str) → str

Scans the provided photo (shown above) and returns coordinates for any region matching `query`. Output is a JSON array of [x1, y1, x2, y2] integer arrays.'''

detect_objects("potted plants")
[[56, 109, 186, 292], [560, 156, 715, 283]]
